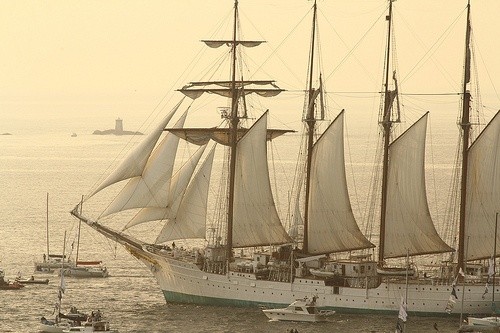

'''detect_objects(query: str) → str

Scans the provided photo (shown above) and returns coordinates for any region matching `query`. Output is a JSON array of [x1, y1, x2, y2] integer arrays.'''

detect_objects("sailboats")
[[69, 0, 500, 318], [468, 212, 500, 333], [34, 192, 103, 273], [58, 194, 109, 278], [40, 231, 68, 332]]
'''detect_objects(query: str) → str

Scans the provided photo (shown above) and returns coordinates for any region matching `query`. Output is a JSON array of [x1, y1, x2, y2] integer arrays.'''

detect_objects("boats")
[[63, 320, 119, 333], [59, 306, 88, 321], [262, 296, 336, 323], [0, 271, 26, 290], [15, 275, 49, 284]]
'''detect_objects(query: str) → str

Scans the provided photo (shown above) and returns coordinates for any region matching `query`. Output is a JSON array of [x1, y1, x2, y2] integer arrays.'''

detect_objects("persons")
[[310, 297, 316, 314]]
